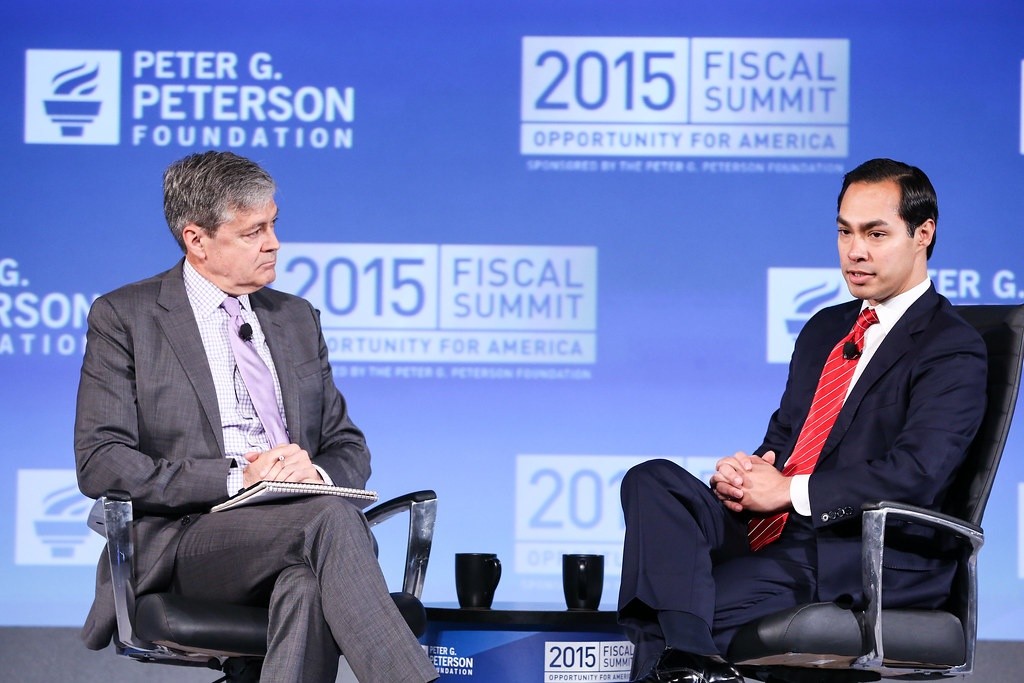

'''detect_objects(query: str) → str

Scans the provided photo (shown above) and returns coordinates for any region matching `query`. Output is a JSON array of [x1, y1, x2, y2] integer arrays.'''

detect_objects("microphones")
[[842, 341, 862, 359], [239, 322, 252, 341]]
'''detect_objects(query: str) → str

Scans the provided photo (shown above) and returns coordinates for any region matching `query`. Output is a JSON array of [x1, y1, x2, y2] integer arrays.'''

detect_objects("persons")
[[73, 151, 441, 683], [618, 158, 989, 682]]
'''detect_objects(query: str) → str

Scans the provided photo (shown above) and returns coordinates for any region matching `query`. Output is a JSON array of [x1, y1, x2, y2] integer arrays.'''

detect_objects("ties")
[[748, 308, 879, 552], [221, 297, 291, 448]]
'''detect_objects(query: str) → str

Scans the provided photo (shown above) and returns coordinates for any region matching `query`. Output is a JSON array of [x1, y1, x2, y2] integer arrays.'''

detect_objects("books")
[[210, 480, 379, 515]]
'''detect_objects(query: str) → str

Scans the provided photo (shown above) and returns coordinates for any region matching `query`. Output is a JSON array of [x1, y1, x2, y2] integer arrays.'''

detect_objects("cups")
[[454, 553, 502, 611], [562, 554, 604, 612]]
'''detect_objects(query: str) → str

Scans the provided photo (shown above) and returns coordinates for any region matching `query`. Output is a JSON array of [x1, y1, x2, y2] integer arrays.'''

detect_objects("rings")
[[278, 456, 285, 469]]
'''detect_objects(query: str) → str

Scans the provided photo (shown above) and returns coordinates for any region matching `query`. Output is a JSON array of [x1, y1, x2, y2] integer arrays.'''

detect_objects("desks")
[[420, 601, 635, 683]]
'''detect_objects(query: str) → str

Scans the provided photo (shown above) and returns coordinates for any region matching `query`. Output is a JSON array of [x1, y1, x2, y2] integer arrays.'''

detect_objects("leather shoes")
[[630, 647, 744, 683]]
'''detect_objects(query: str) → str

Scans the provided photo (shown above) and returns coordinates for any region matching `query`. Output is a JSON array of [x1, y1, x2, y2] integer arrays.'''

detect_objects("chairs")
[[724, 304, 1024, 683], [86, 490, 438, 683]]
